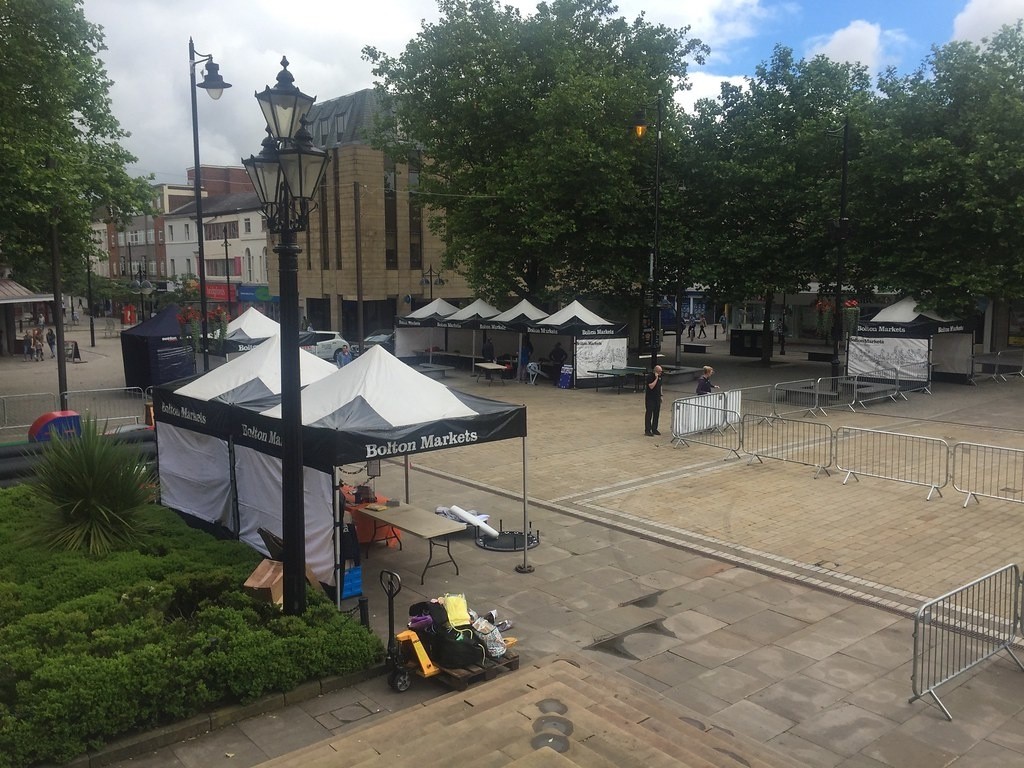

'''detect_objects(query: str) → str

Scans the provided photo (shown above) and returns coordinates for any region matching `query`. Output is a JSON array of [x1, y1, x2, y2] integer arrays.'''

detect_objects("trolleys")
[[380, 569, 519, 694]]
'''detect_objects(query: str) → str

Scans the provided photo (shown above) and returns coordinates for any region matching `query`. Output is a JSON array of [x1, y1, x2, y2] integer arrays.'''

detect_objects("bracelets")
[[661, 395, 663, 396]]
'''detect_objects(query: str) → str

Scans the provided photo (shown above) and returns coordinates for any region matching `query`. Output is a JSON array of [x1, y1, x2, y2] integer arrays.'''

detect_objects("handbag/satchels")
[[406, 591, 518, 669]]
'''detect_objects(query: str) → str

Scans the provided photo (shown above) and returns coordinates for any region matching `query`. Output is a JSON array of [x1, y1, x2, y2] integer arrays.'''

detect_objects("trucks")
[[658, 299, 688, 335]]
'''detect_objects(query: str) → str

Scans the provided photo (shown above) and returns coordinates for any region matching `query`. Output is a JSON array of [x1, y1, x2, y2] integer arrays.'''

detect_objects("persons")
[[549, 343, 568, 386], [645, 365, 664, 437], [719, 312, 726, 333], [687, 314, 707, 338], [696, 366, 720, 430], [337, 345, 352, 368], [482, 337, 496, 379], [38, 313, 45, 330], [24, 328, 56, 362], [308, 323, 313, 332], [78, 298, 83, 309], [517, 339, 531, 380]]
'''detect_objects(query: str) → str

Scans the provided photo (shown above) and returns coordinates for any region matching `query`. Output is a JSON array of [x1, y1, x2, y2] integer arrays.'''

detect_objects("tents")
[[120, 303, 317, 393], [153, 335, 527, 612], [847, 295, 975, 393], [394, 297, 629, 389]]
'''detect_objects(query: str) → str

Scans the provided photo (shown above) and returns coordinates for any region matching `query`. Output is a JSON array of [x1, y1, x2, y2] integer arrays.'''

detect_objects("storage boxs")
[[339, 562, 362, 599], [243, 559, 283, 610]]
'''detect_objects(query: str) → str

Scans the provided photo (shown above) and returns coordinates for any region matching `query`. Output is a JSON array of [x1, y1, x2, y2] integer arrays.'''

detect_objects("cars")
[[351, 328, 394, 358]]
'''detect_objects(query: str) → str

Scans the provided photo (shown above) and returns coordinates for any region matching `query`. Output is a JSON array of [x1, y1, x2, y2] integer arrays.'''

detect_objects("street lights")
[[79, 190, 103, 347], [633, 89, 666, 366], [241, 54, 331, 616], [420, 262, 446, 303], [128, 261, 154, 322], [186, 37, 234, 370], [827, 108, 849, 390]]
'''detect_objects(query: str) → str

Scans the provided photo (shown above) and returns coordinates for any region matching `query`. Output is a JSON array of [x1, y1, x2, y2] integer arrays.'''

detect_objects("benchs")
[[680, 343, 711, 353], [416, 363, 455, 378], [777, 380, 901, 407], [631, 365, 703, 385], [802, 350, 845, 362]]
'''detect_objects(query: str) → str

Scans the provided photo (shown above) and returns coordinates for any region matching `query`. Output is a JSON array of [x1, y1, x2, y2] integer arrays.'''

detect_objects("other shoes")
[[704, 336, 707, 338], [51, 356, 53, 358], [652, 430, 660, 435], [553, 383, 556, 386], [722, 331, 725, 334], [645, 431, 654, 436], [36, 359, 38, 362], [53, 355, 55, 357], [687, 336, 690, 338], [697, 336, 700, 339], [41, 359, 45, 361]]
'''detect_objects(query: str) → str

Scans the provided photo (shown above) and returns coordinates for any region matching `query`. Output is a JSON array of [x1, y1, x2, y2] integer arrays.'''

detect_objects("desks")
[[586, 368, 645, 394], [339, 486, 401, 548], [358, 501, 467, 585], [412, 349, 563, 388]]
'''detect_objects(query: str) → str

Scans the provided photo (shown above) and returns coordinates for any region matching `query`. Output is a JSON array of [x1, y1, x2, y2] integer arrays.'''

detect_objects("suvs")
[[298, 331, 351, 362]]
